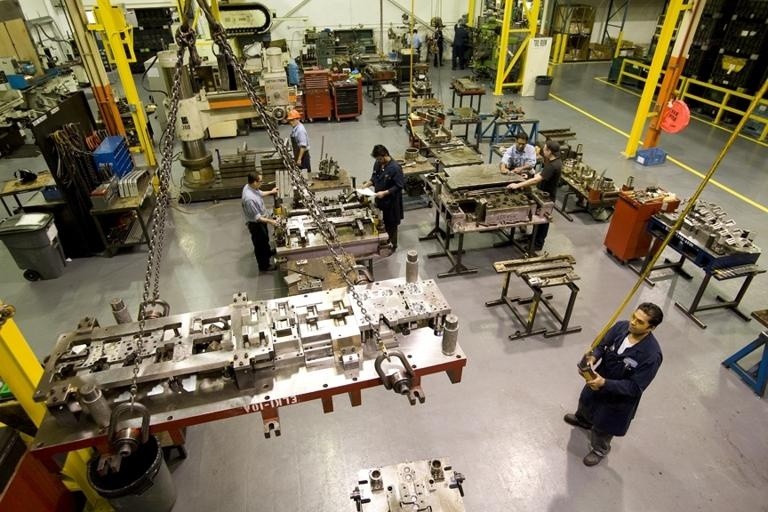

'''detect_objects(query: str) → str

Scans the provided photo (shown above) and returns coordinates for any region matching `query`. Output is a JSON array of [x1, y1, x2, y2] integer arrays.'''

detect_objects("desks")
[[87, 165, 161, 257], [1, 167, 60, 216]]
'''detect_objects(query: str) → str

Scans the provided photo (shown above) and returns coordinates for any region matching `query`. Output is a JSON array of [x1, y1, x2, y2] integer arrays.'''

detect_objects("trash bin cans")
[[87, 432, 177, 512], [535, 75, 553, 100], [0, 212, 67, 281]]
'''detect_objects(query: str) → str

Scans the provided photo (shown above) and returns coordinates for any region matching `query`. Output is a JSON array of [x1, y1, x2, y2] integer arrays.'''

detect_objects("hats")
[[286, 110, 302, 120], [546, 141, 562, 156]]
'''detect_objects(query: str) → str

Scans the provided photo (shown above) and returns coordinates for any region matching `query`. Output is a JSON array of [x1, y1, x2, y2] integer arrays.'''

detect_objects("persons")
[[241, 170, 278, 272], [434, 24, 446, 67], [287, 109, 312, 172], [359, 144, 408, 257], [507, 140, 561, 250], [500, 132, 537, 233], [564, 302, 663, 467], [409, 29, 420, 49], [451, 23, 468, 70]]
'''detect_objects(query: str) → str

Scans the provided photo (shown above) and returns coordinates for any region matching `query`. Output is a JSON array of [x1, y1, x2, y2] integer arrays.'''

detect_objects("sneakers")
[[564, 413, 592, 429], [583, 447, 612, 467], [524, 244, 543, 251], [259, 264, 277, 272], [519, 225, 527, 234]]
